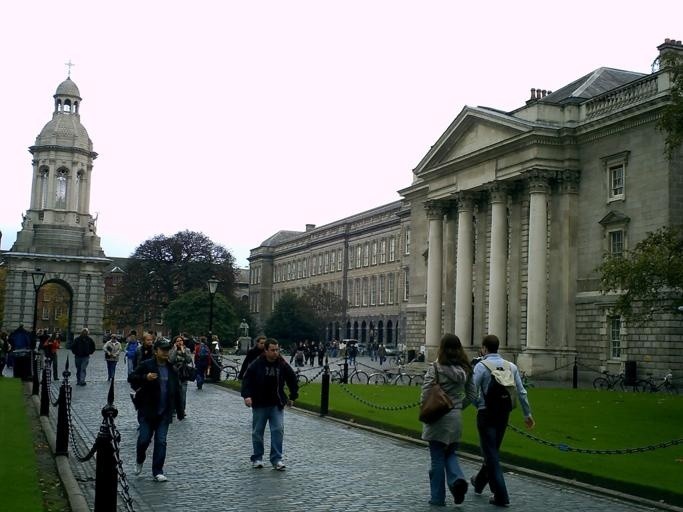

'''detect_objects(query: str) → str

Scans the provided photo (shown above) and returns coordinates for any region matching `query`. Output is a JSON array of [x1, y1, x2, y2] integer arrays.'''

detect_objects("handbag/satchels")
[[179, 355, 198, 382], [418, 361, 453, 426]]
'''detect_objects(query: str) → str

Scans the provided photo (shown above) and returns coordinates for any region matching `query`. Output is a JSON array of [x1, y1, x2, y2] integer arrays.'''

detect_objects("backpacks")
[[479, 359, 518, 417]]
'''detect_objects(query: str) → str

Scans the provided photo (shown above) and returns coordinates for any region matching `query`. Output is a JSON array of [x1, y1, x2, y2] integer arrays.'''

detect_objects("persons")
[[378, 344, 386, 366], [304, 339, 311, 364], [237, 336, 267, 382], [295, 342, 305, 367], [241, 338, 300, 471], [289, 338, 299, 364], [194, 336, 210, 391], [327, 337, 358, 366], [372, 341, 379, 362], [418, 333, 475, 504], [208, 334, 223, 383], [182, 332, 195, 354], [133, 333, 154, 372], [38, 328, 50, 369], [43, 332, 60, 380], [169, 336, 192, 417], [309, 341, 318, 368], [124, 331, 142, 375], [471, 334, 536, 508], [7, 321, 28, 368], [127, 339, 186, 482], [148, 330, 169, 345], [71, 328, 95, 386], [102, 333, 122, 381], [317, 341, 327, 366], [0, 329, 11, 376]]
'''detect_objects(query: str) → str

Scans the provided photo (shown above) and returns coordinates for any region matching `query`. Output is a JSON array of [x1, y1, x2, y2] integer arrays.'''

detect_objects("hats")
[[154, 339, 171, 350]]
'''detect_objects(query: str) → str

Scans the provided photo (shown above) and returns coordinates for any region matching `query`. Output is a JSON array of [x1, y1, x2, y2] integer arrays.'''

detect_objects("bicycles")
[[632, 369, 679, 393], [216, 356, 241, 382], [369, 364, 411, 385], [389, 352, 406, 368], [412, 367, 428, 384], [331, 360, 368, 384], [593, 371, 630, 392]]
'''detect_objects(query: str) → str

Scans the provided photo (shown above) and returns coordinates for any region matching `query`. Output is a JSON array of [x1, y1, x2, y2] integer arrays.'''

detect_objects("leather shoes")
[[453, 481, 469, 505], [470, 474, 483, 495], [427, 499, 447, 507], [487, 495, 511, 506]]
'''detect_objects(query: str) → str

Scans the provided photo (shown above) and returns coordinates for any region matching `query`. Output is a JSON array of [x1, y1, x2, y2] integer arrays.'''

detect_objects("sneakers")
[[152, 473, 168, 482], [133, 462, 143, 475], [271, 462, 286, 470], [251, 460, 264, 468]]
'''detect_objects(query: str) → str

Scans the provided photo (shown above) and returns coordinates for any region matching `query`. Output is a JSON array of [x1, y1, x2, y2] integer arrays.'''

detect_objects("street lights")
[[207, 275, 219, 348], [31, 266, 46, 332]]
